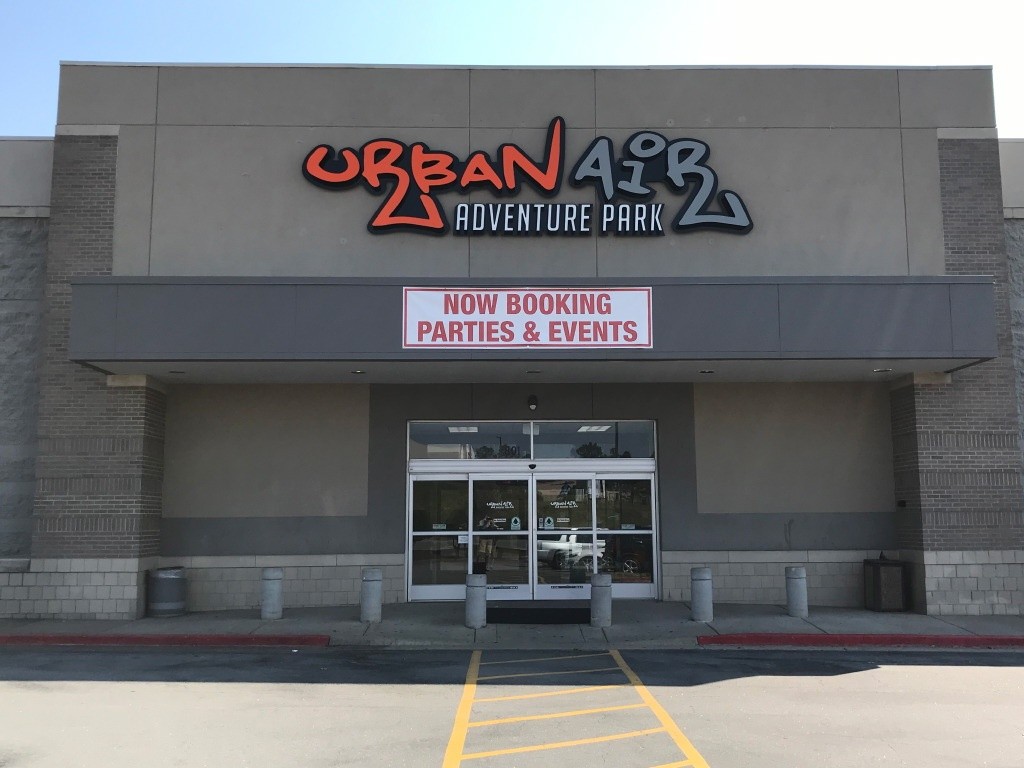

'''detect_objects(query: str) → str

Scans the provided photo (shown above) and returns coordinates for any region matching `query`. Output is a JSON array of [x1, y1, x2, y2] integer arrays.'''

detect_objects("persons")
[[476, 515, 499, 572]]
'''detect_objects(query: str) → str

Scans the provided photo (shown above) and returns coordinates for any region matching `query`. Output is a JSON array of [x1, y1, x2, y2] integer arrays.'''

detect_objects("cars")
[[537, 526, 652, 575]]
